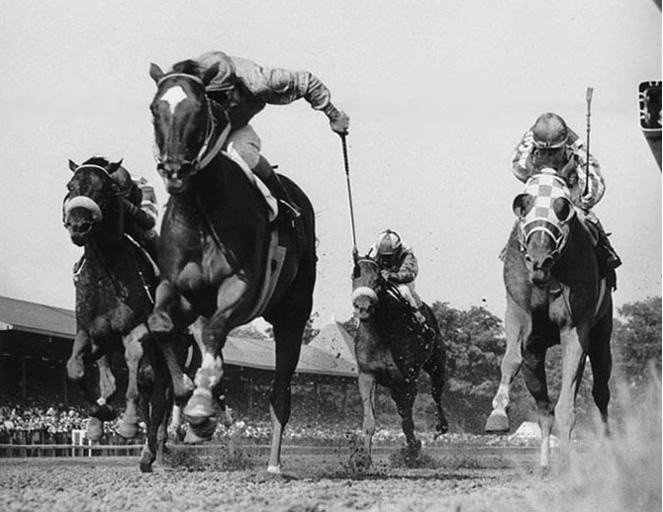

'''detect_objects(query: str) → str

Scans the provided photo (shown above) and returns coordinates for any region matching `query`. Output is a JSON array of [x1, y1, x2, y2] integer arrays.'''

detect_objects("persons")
[[2, 396, 257, 455], [82, 157, 162, 258], [366, 230, 424, 324], [171, 51, 350, 219], [513, 113, 606, 213]]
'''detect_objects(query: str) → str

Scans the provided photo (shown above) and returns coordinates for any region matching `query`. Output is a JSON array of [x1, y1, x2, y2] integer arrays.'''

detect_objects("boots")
[[266, 169, 304, 223], [594, 219, 621, 268]]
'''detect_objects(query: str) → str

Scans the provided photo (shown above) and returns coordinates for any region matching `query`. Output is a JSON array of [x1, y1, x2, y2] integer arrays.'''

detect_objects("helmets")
[[531, 113, 569, 151], [110, 164, 135, 195], [375, 228, 401, 255], [196, 51, 235, 92]]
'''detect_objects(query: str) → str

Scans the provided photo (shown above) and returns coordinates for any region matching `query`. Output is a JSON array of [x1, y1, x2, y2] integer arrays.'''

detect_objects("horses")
[[351, 245, 449, 472], [483, 153, 614, 480], [145, 61, 318, 476], [62, 156, 234, 443]]
